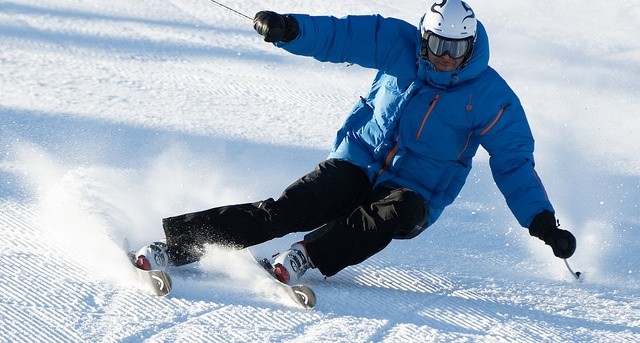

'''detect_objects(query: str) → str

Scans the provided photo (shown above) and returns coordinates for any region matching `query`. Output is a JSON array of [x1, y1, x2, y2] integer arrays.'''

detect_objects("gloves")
[[253, 11, 298, 42], [529, 211, 576, 258]]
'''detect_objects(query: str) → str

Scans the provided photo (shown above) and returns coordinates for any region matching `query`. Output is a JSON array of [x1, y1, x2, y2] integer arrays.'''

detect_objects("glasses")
[[428, 35, 473, 59]]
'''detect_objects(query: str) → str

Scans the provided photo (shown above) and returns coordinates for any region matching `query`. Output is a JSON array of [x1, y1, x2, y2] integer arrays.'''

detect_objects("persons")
[[135, 0, 576, 283]]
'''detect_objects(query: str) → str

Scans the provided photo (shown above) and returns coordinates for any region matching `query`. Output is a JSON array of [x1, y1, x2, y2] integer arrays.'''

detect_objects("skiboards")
[[125, 237, 317, 311]]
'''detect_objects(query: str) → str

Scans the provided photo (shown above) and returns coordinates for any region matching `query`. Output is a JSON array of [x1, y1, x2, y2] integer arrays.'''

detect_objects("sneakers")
[[134, 242, 169, 270], [272, 241, 311, 283]]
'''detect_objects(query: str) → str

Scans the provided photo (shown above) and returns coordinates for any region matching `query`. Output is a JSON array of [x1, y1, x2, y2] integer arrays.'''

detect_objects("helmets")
[[421, 0, 478, 39]]
